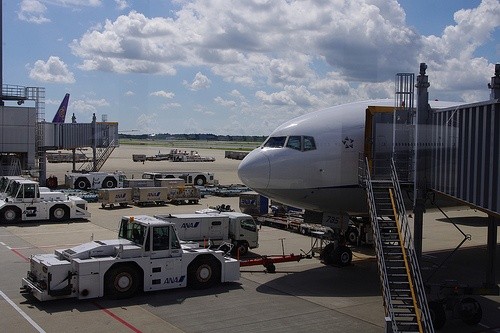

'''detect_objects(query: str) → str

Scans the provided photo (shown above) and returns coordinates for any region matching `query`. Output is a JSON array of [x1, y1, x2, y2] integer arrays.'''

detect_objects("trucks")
[[0, 175, 91, 227], [152, 210, 262, 257]]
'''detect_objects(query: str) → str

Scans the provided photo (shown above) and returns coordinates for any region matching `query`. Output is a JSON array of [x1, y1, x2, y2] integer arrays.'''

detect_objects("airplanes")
[[237, 97, 474, 217]]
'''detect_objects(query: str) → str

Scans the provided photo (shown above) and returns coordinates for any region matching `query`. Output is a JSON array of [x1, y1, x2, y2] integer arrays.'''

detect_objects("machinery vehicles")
[[19, 214, 240, 303]]
